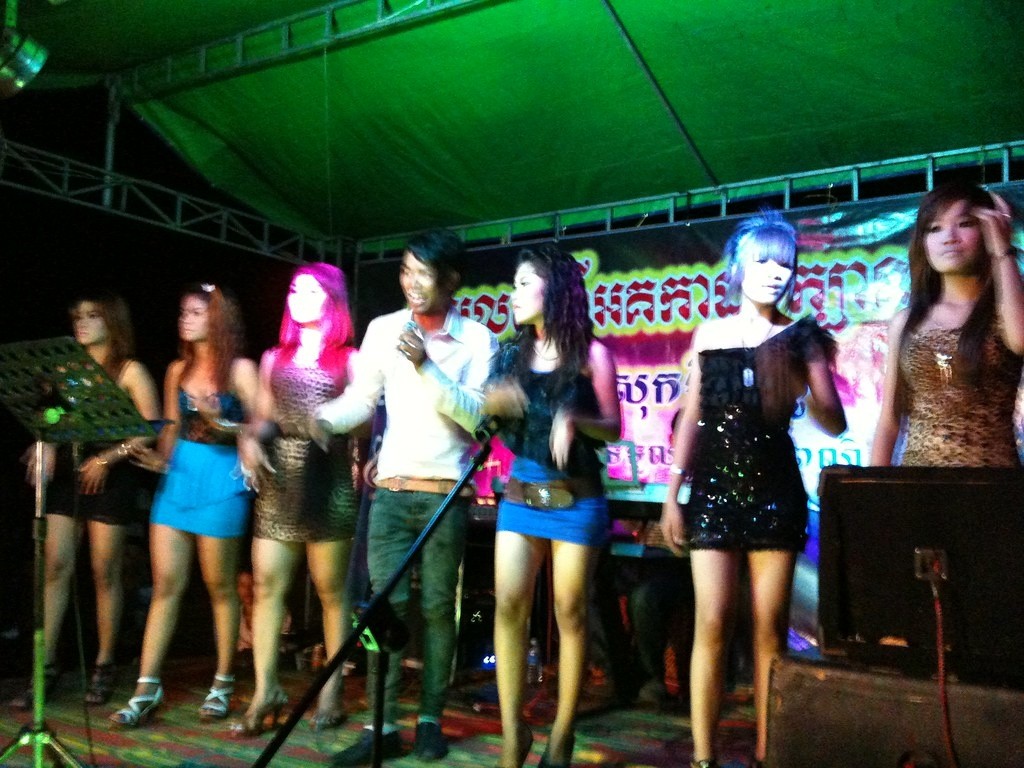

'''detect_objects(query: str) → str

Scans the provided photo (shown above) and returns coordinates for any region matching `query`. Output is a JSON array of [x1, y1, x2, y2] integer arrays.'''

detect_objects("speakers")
[[818, 464, 1024, 693], [764, 657, 1024, 768]]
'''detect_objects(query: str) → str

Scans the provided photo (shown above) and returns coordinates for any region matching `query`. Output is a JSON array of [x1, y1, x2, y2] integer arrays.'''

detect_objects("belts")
[[504, 478, 606, 508], [376, 477, 474, 497]]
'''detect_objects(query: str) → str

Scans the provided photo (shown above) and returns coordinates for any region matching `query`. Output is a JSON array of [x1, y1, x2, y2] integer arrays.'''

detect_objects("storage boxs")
[[766, 645, 1024, 768]]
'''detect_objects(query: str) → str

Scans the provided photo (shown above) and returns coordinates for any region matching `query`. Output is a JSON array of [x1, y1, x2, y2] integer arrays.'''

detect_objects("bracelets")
[[668, 466, 686, 476], [989, 248, 1021, 259]]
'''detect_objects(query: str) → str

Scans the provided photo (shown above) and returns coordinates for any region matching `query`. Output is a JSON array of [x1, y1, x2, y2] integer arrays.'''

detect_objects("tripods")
[[0, 384, 88, 768]]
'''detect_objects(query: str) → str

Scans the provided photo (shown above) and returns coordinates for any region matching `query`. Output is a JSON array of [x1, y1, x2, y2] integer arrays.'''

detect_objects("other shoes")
[[538, 731, 575, 768], [497, 719, 535, 768], [748, 749, 771, 768], [689, 751, 717, 768], [330, 727, 402, 768], [414, 719, 448, 762]]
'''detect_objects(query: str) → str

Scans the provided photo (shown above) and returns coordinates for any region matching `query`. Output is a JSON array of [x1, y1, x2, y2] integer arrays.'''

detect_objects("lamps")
[[0, 0, 49, 103]]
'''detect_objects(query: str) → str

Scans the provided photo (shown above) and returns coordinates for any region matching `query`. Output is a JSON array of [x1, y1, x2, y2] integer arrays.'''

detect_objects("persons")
[[866, 180, 1023, 469], [661, 213, 849, 768], [10, 294, 160, 710], [234, 264, 364, 740], [109, 285, 261, 737], [483, 245, 622, 768], [307, 230, 501, 767]]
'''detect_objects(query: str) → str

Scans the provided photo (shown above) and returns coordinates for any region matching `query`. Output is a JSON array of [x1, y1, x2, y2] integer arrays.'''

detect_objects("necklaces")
[[524, 328, 563, 363], [735, 306, 777, 388], [932, 291, 981, 382]]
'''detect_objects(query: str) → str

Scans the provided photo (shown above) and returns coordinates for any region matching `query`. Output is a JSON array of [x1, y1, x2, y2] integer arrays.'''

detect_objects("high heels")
[[108, 676, 164, 726], [226, 683, 288, 738], [308, 670, 348, 731], [197, 671, 237, 719]]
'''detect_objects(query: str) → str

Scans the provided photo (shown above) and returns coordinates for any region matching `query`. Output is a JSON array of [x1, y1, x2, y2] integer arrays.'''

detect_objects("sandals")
[[84, 661, 117, 706], [7, 669, 56, 709]]
